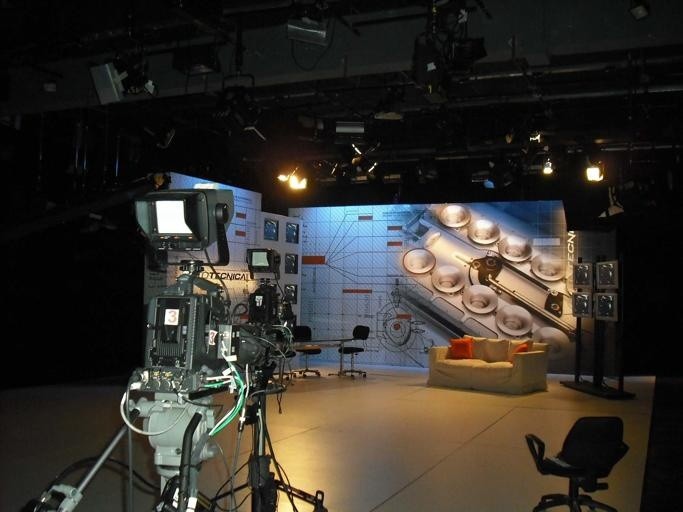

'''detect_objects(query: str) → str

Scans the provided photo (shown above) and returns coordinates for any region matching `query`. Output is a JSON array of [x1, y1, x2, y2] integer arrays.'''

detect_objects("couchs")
[[426, 343, 551, 395]]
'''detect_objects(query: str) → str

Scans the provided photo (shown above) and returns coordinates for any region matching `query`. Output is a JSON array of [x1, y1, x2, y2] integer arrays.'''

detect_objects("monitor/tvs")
[[572, 262, 595, 289]]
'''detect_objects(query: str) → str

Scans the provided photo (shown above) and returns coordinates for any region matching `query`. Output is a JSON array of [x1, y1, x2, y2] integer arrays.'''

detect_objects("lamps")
[[274, 143, 380, 192], [541, 150, 606, 183]]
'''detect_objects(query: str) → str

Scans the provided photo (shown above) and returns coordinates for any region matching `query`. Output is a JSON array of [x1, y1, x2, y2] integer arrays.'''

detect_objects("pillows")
[[448, 333, 533, 363]]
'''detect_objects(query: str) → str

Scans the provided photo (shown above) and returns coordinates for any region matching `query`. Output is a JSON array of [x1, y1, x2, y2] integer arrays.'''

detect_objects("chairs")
[[524, 416, 630, 512], [266, 329, 296, 380], [338, 325, 370, 378], [292, 324, 321, 377]]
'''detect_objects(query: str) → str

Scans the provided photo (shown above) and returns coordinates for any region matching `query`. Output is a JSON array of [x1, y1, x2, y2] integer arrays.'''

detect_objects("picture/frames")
[[573, 263, 594, 289]]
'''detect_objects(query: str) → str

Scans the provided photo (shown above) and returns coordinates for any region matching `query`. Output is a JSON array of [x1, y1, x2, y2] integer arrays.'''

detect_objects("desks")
[[293, 337, 353, 378]]
